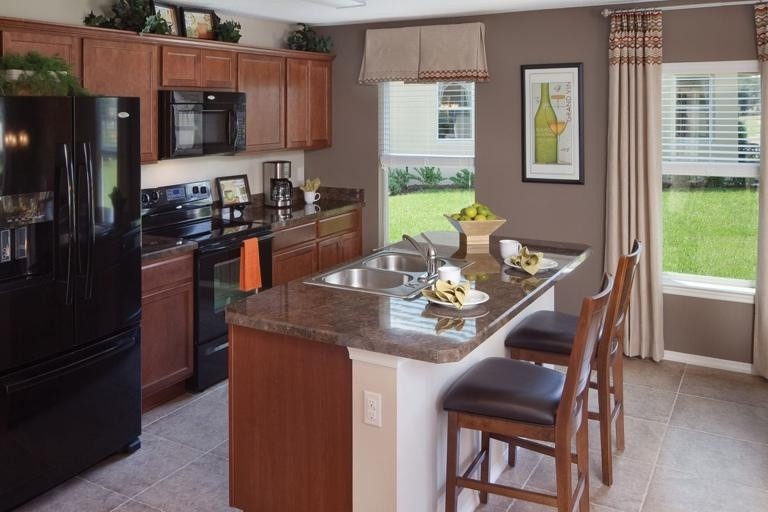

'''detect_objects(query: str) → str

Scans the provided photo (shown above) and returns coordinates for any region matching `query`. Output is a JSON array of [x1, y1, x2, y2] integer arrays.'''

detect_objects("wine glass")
[[545, 105, 568, 165]]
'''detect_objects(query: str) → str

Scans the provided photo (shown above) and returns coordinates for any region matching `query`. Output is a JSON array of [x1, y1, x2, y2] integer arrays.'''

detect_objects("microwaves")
[[160, 91, 246, 159]]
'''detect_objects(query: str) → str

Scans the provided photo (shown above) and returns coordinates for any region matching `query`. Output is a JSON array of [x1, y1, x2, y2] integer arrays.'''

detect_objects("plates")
[[426, 289, 489, 309], [504, 257, 558, 272]]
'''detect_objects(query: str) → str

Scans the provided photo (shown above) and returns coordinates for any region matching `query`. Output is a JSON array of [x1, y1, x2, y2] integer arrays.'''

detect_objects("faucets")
[[401, 232, 438, 276]]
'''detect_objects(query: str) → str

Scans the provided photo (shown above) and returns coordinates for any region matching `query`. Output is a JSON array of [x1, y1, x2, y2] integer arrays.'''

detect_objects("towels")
[[298, 176, 321, 192], [420, 278, 470, 310], [510, 246, 544, 277], [238, 237, 263, 292]]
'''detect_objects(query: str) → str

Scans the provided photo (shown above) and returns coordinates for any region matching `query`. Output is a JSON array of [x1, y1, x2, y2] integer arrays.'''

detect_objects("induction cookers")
[[142, 217, 271, 242]]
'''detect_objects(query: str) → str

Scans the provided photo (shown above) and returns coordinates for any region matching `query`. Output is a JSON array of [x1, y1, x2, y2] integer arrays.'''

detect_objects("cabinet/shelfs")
[[286, 48, 337, 151], [0, 16, 80, 101], [82, 26, 160, 166], [159, 34, 237, 92], [235, 45, 287, 154], [271, 202, 363, 290], [140, 242, 198, 413]]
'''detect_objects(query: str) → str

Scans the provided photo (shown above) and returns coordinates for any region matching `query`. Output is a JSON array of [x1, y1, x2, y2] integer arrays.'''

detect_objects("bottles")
[[535, 82, 557, 165]]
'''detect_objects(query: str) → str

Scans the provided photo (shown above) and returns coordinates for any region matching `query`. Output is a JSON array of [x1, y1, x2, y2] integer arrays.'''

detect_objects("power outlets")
[[363, 389, 383, 429]]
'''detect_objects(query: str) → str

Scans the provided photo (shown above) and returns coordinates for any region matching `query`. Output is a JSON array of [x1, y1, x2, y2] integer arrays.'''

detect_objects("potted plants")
[[211, 17, 243, 43], [0, 49, 93, 97]]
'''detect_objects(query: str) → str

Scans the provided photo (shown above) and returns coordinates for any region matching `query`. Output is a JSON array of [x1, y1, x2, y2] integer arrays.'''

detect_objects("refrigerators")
[[0, 94, 142, 511]]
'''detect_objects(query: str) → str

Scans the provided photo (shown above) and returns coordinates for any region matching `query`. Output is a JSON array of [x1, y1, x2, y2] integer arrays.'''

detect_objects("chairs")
[[442, 270, 615, 512], [504, 238, 644, 487]]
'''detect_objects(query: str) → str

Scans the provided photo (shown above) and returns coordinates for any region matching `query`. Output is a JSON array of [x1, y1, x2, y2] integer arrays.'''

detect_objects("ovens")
[[194, 234, 272, 392]]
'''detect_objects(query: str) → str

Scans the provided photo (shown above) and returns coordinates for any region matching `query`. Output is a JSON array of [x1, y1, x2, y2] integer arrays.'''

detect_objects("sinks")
[[355, 245, 476, 273], [302, 262, 435, 299]]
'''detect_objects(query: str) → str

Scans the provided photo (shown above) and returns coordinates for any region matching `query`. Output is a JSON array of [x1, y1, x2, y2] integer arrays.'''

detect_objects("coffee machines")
[[263, 160, 293, 207]]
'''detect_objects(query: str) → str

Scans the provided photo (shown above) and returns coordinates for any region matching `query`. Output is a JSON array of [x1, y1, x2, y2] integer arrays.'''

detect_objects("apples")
[[452, 201, 498, 221]]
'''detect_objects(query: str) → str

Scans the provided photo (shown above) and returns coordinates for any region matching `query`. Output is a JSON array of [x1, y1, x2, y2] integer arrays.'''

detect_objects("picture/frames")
[[215, 173, 253, 207], [178, 6, 215, 39], [149, 0, 180, 36], [520, 62, 585, 185]]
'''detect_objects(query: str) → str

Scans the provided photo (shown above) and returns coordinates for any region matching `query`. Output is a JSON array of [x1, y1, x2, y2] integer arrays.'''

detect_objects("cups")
[[438, 266, 461, 283], [304, 192, 320, 203], [499, 240, 522, 259]]
